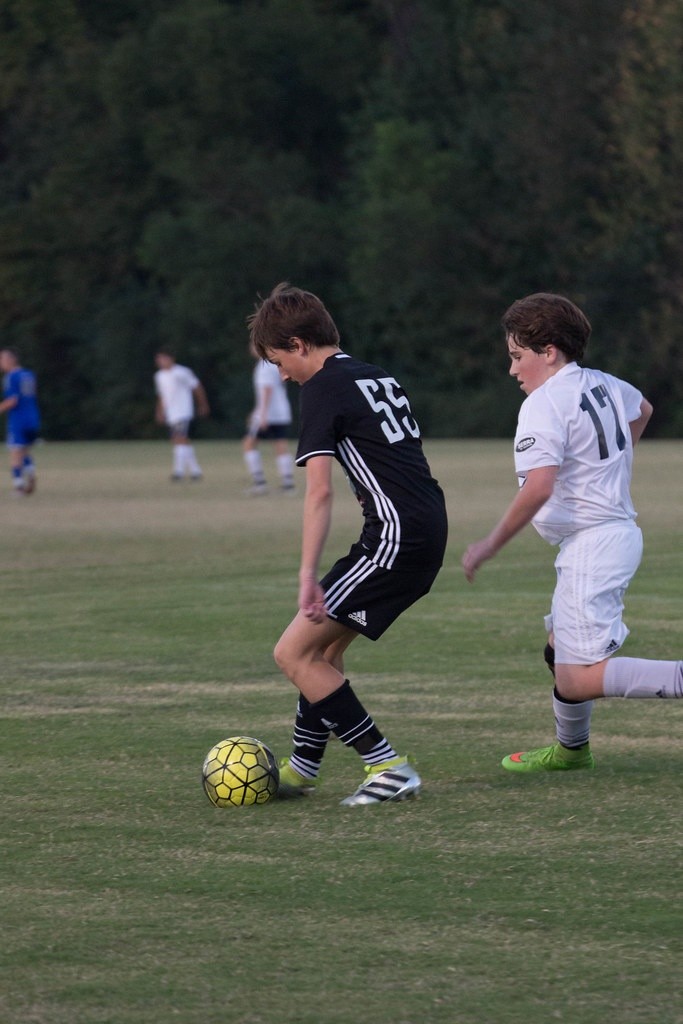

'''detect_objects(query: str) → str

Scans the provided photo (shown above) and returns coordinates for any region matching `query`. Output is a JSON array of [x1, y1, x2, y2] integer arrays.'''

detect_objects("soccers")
[[200, 736, 279, 808]]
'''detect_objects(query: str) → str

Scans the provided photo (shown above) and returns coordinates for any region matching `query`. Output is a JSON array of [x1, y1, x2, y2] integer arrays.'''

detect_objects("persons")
[[244, 282, 450, 805], [154, 352, 210, 481], [0, 350, 43, 493], [242, 343, 298, 493], [458, 294, 683, 776]]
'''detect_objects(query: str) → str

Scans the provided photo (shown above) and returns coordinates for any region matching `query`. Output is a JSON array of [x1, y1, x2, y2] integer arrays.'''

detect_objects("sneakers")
[[338, 756, 422, 808], [273, 758, 322, 793], [501, 739, 595, 771]]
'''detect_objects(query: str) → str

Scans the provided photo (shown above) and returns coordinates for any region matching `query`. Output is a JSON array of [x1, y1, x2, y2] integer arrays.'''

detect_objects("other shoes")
[[172, 469, 202, 481], [281, 482, 298, 493], [15, 467, 36, 496], [245, 480, 269, 496]]
